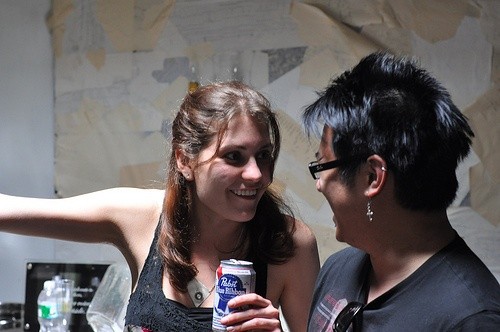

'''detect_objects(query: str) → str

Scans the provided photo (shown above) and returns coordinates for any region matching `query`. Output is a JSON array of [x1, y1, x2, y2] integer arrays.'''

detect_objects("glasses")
[[332, 301, 363, 332], [309, 154, 393, 180]]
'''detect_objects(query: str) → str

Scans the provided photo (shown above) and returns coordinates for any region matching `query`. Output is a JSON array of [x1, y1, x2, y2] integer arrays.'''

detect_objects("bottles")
[[37, 276, 74, 332]]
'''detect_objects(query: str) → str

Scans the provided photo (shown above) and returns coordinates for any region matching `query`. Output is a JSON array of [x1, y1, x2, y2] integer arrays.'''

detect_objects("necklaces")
[[186, 241, 244, 308]]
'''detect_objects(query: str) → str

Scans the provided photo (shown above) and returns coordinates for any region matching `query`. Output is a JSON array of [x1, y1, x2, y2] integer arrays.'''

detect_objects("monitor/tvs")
[[23, 262, 111, 332]]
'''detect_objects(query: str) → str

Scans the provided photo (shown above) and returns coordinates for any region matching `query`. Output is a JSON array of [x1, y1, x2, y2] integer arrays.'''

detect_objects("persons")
[[301, 49, 500, 332], [0, 80, 320, 332]]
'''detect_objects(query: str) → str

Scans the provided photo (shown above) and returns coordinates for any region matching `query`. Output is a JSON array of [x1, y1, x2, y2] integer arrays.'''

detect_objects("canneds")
[[212, 258, 256, 332]]
